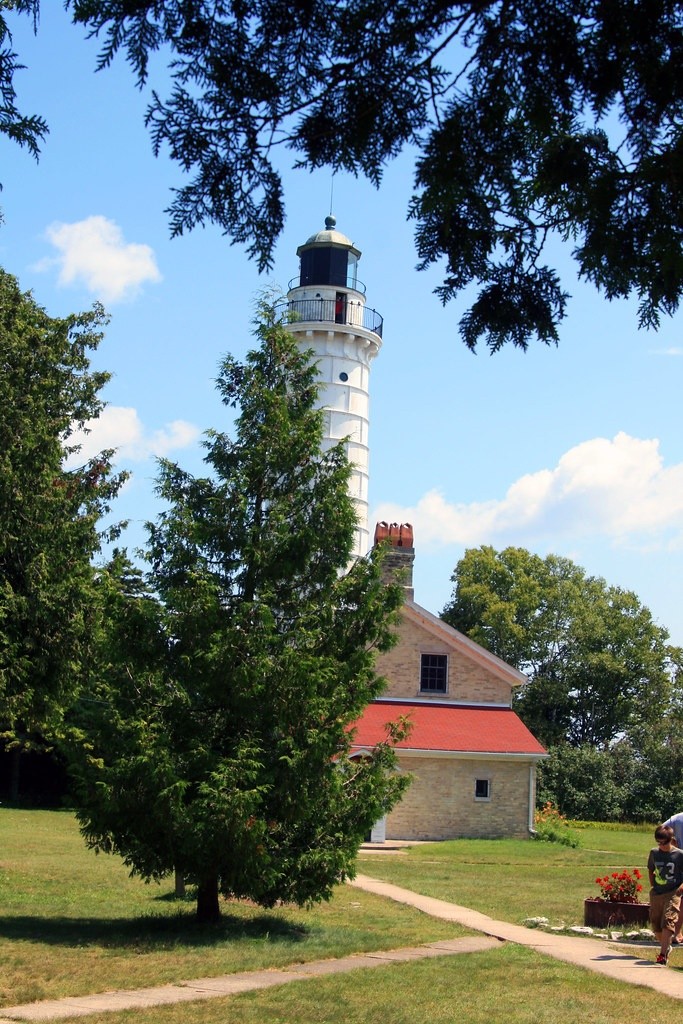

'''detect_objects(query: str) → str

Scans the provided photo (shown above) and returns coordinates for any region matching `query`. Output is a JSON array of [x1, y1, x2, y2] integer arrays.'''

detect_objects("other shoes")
[[672, 933, 682, 944]]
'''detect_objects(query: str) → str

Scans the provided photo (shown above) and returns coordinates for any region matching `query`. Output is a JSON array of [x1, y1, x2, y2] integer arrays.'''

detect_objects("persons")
[[647, 812, 683, 967], [336, 295, 344, 323]]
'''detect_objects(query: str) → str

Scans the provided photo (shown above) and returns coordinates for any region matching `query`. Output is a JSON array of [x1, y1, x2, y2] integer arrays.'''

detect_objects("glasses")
[[657, 840, 671, 845]]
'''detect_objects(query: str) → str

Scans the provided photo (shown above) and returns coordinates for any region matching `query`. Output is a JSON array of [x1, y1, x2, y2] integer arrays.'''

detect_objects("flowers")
[[595, 869, 643, 903]]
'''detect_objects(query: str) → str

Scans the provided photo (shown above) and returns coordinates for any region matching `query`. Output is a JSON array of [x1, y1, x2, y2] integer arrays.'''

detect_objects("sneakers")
[[654, 952, 667, 968], [665, 945, 672, 963]]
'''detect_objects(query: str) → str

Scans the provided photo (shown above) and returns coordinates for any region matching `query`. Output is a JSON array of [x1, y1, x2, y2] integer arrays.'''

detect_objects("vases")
[[585, 898, 650, 929]]
